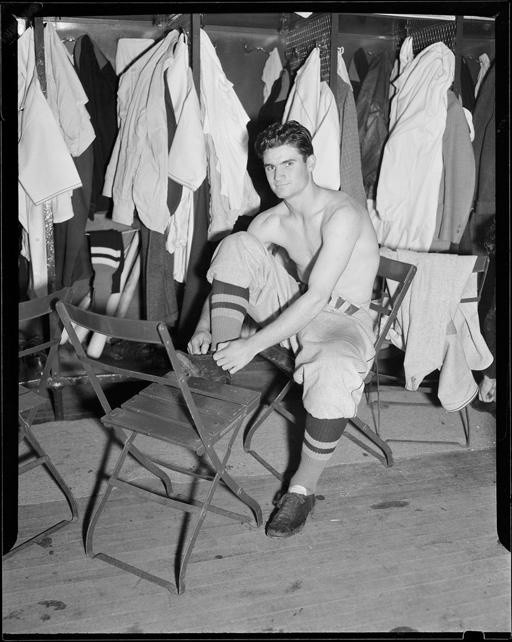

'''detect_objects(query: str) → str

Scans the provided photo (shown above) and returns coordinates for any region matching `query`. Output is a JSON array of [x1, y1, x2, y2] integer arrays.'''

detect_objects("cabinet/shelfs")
[[10, 8, 501, 423]]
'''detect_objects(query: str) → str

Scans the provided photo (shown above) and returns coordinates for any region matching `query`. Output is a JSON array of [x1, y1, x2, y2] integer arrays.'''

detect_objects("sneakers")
[[263, 487, 318, 540], [173, 349, 233, 386]]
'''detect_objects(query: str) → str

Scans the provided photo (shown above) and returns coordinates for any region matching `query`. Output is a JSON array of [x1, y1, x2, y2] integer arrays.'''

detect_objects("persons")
[[185, 119, 381, 538]]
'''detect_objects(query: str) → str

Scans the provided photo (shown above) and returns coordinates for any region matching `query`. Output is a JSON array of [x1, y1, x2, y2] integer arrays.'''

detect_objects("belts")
[[328, 297, 359, 317]]
